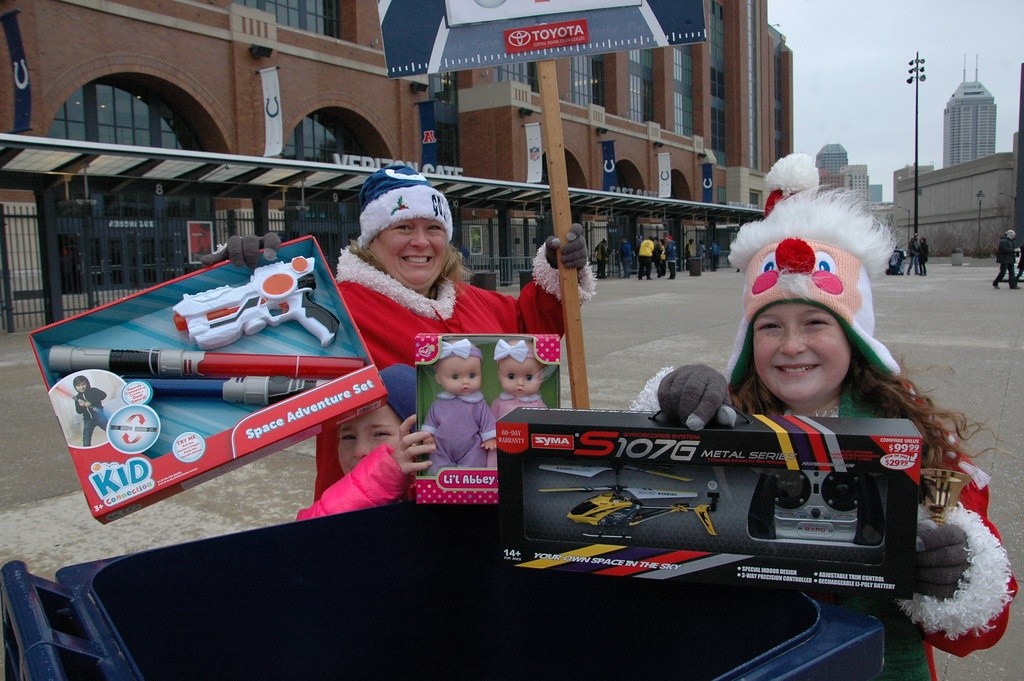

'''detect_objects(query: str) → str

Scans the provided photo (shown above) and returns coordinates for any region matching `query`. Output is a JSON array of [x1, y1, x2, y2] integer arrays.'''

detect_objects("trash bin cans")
[[951, 248, 963, 266], [517, 270, 533, 291], [688, 258, 703, 276], [0, 499, 888, 681], [469, 272, 496, 291]]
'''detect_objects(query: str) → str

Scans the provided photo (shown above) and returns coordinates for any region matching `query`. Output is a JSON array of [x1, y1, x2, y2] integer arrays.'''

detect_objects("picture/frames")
[[187, 220, 214, 265], [468, 225, 484, 255]]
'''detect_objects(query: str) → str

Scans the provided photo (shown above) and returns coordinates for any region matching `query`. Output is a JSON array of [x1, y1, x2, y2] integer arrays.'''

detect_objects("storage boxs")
[[414, 332, 562, 506], [493, 408, 924, 602], [26, 234, 389, 524]]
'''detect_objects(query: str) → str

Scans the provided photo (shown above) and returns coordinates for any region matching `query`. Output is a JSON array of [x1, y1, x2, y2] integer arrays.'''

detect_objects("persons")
[[629, 155, 1019, 681], [992, 229, 1024, 289], [907, 233, 928, 276], [72, 376, 109, 447], [421, 338, 558, 476], [594, 235, 719, 280], [297, 363, 436, 521], [200, 166, 598, 500]]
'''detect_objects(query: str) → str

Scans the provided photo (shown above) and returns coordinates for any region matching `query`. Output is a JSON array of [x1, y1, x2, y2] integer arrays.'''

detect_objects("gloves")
[[545, 224, 587, 274], [657, 364, 738, 432], [199, 233, 281, 271], [912, 520, 971, 601]]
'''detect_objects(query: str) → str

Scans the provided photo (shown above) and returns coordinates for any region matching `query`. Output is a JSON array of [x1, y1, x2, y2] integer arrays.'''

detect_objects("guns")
[[170, 254, 342, 350]]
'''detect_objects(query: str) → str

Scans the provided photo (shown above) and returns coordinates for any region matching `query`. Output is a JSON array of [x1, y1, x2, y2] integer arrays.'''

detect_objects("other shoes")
[[1010, 285, 1021, 289], [915, 272, 919, 275], [994, 285, 1000, 289], [907, 272, 910, 275]]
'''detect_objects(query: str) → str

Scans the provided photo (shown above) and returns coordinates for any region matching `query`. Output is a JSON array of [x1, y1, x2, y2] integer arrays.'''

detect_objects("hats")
[[725, 151, 901, 388], [358, 166, 453, 248], [379, 364, 417, 434]]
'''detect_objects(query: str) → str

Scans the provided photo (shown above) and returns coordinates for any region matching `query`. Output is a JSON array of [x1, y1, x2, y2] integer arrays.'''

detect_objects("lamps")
[[248, 43, 273, 61], [518, 107, 534, 118], [409, 80, 429, 93], [480, 70, 489, 78], [654, 141, 663, 147], [371, 38, 379, 47], [698, 153, 707, 158], [596, 127, 608, 135]]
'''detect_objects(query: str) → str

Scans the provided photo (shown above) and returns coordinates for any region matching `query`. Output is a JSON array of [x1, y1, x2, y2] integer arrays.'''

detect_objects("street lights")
[[909, 51, 926, 234], [976, 191, 984, 246], [897, 206, 910, 244]]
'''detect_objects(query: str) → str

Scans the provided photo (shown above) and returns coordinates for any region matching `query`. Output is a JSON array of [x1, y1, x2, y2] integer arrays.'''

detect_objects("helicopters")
[[538, 459, 720, 539]]
